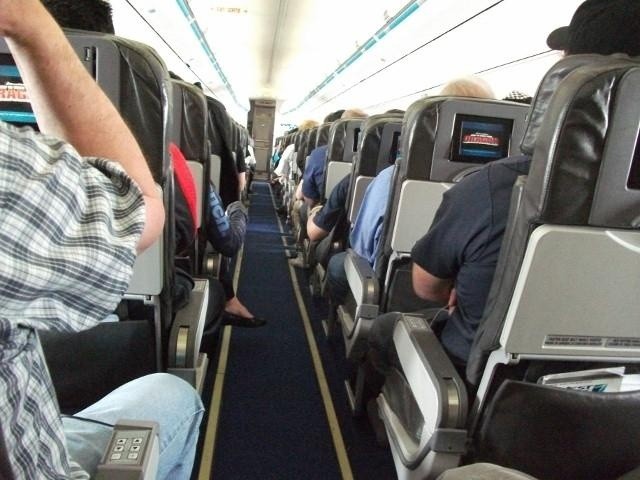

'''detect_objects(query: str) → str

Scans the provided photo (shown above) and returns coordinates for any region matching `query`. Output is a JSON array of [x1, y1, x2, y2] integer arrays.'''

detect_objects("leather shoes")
[[221, 311, 268, 328]]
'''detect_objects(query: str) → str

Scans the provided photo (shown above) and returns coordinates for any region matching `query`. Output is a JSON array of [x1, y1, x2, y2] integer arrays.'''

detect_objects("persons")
[[1, 0, 256, 480], [353, 1, 640, 451], [270, 79, 533, 310]]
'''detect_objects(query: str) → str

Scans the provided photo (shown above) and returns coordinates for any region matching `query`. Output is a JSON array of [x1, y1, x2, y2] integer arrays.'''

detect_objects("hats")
[[546, 1, 640, 56]]
